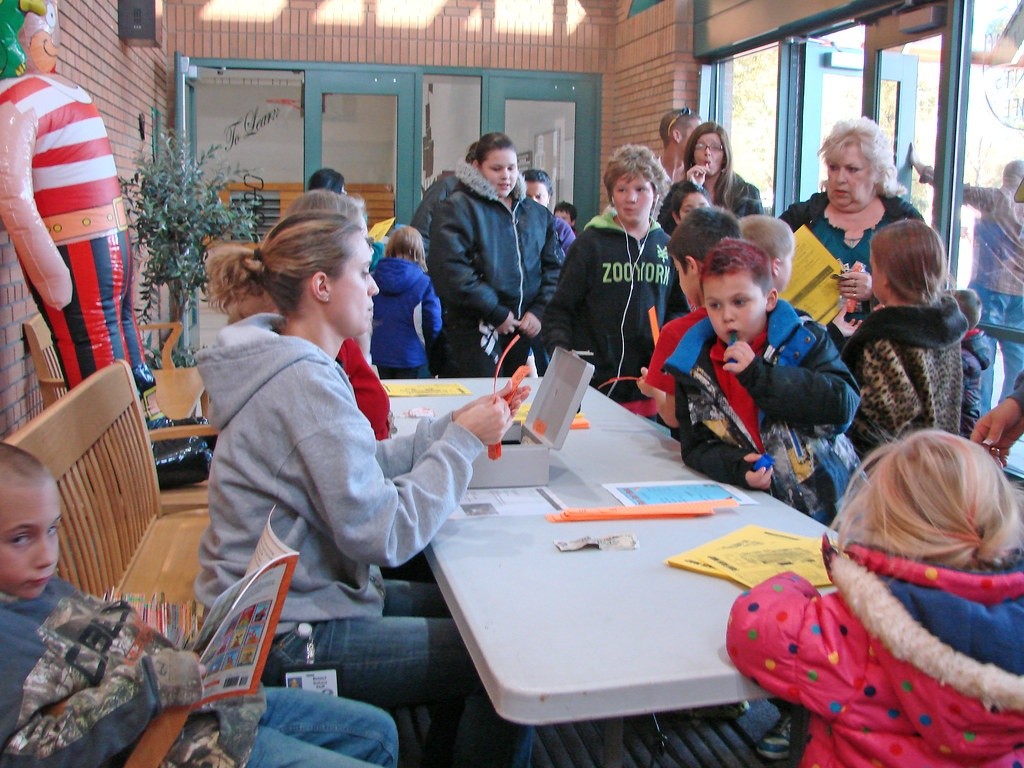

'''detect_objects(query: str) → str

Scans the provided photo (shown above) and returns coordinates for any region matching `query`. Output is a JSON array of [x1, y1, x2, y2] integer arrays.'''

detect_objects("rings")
[[853, 279, 856, 287], [852, 294, 856, 299], [991, 447, 1000, 451], [853, 288, 856, 294]]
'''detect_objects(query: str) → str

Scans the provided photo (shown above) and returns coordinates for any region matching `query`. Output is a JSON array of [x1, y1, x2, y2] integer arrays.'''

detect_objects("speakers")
[[118, 0, 163, 48]]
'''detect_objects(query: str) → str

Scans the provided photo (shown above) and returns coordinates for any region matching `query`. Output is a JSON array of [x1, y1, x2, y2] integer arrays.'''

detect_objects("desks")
[[383, 376, 840, 768]]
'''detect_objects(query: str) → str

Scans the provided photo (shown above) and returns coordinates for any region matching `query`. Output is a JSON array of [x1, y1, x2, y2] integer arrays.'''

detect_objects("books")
[[104, 587, 198, 652], [192, 505, 300, 709]]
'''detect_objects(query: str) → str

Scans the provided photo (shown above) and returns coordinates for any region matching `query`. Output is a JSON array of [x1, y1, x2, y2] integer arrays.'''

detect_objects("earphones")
[[611, 196, 615, 205], [656, 194, 659, 201]]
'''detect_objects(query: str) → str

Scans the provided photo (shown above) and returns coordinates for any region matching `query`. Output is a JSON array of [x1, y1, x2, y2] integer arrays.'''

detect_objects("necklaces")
[[845, 238, 862, 247]]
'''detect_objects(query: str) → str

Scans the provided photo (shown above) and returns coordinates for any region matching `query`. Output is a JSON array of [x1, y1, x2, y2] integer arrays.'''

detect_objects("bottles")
[[278, 623, 315, 666]]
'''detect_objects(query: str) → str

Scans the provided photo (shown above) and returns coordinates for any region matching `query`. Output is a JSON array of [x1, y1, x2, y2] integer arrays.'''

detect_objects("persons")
[[778, 116, 927, 343], [840, 220, 969, 478], [0, 441, 399, 768], [656, 108, 764, 325], [726, 430, 1024, 768], [371, 226, 442, 380], [911, 144, 1024, 467], [192, 189, 534, 768], [636, 205, 794, 444], [660, 238, 868, 759], [308, 133, 692, 403], [290, 679, 301, 690]]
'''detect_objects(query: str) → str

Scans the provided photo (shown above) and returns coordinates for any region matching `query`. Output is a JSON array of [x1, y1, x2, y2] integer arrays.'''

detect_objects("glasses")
[[696, 143, 723, 152], [668, 106, 691, 137]]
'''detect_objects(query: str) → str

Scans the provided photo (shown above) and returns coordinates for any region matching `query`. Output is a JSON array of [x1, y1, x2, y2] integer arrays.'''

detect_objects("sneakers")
[[756, 713, 791, 760]]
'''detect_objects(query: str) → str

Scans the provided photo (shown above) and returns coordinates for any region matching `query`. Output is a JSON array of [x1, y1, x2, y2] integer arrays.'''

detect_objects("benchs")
[[3, 359, 219, 653], [22, 313, 208, 421]]
